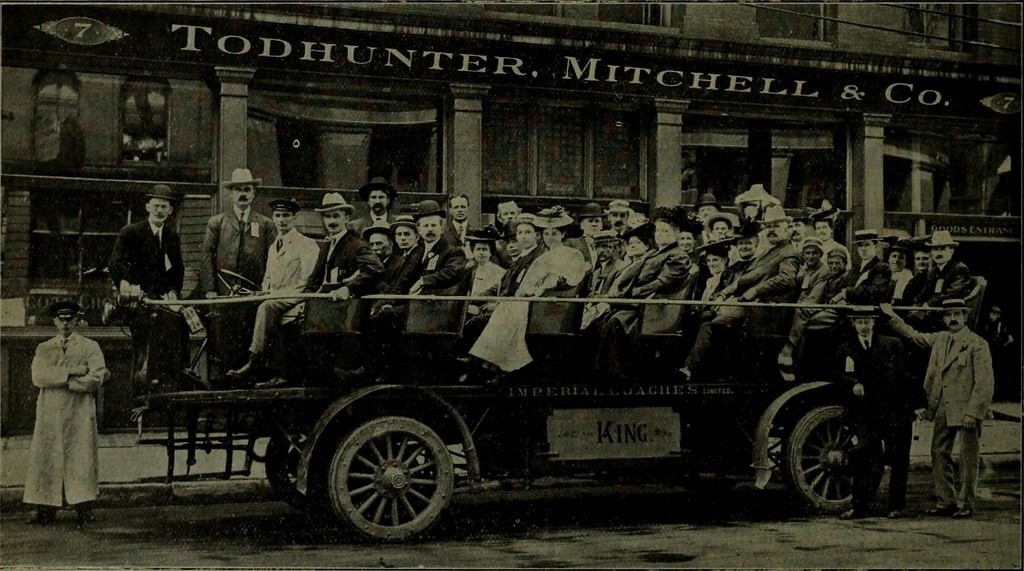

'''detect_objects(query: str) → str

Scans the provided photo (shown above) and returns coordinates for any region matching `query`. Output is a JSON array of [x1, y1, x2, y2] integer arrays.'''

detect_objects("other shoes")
[[838, 507, 868, 520], [678, 367, 692, 382], [456, 356, 477, 367], [25, 511, 56, 524], [487, 372, 509, 387], [887, 509, 906, 519], [254, 377, 288, 390], [952, 509, 973, 519], [225, 361, 262, 380], [71, 512, 96, 531], [925, 506, 959, 517]]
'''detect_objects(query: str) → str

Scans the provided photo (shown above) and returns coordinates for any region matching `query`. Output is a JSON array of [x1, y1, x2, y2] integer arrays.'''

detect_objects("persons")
[[985, 306, 1014, 350], [108, 184, 189, 370], [879, 299, 995, 519], [200, 167, 976, 387], [22, 301, 106, 524], [832, 306, 912, 519]]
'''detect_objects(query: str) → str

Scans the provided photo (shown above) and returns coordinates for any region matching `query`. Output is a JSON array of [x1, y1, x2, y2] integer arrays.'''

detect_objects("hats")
[[936, 297, 974, 314], [222, 167, 263, 190], [390, 213, 418, 233], [49, 298, 81, 320], [412, 199, 447, 222], [846, 305, 880, 319], [269, 194, 301, 215], [358, 176, 396, 203], [462, 191, 961, 265], [314, 191, 355, 216], [362, 219, 393, 243], [145, 184, 176, 201]]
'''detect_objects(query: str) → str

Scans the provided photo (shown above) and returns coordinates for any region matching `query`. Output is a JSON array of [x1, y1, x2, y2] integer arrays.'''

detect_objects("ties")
[[864, 340, 871, 351]]
